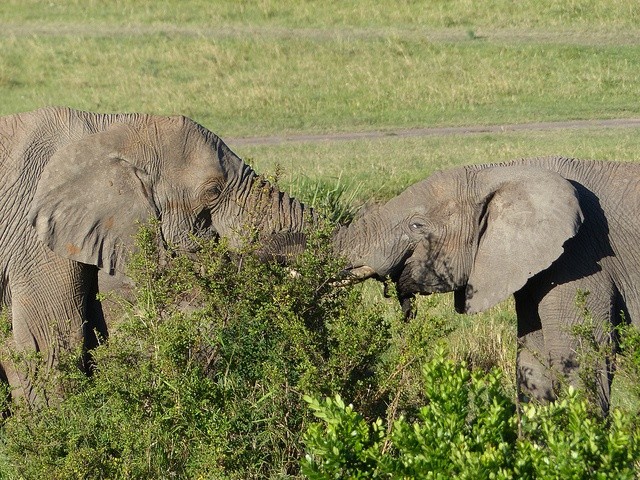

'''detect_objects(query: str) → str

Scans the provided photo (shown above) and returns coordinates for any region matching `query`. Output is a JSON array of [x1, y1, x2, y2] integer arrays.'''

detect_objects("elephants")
[[0, 105, 341, 445], [247, 154, 640, 437]]
